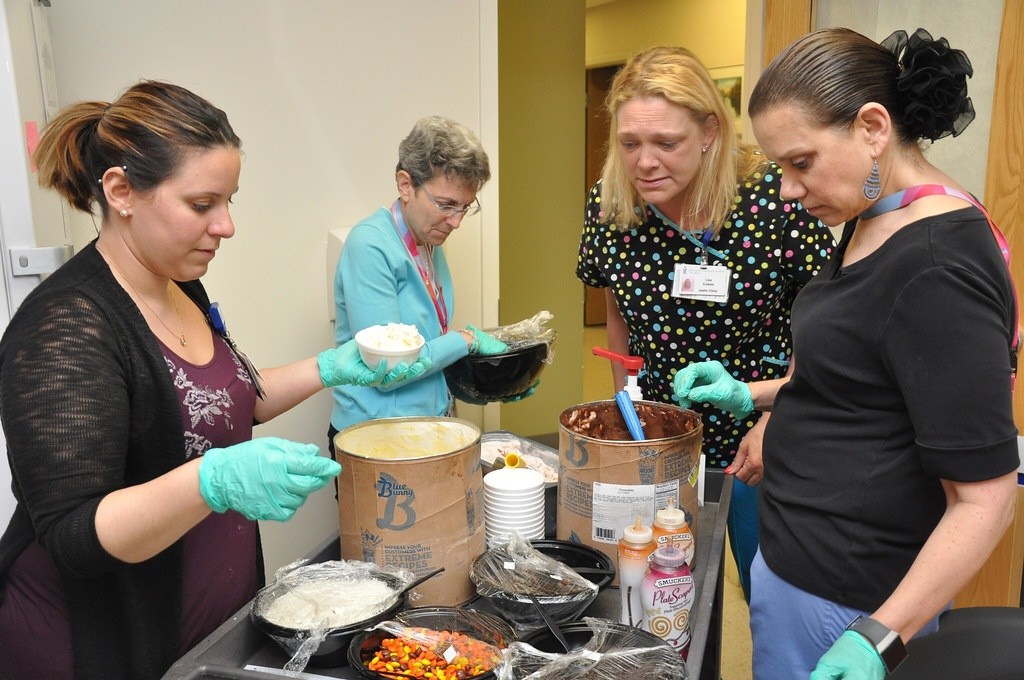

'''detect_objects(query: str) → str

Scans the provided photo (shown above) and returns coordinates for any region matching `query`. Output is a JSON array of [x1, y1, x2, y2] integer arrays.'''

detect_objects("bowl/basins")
[[443, 325, 548, 400], [345, 606, 517, 680], [249, 560, 409, 665], [468, 538, 615, 623], [355, 326, 425, 373], [509, 621, 687, 680], [483, 468, 546, 551]]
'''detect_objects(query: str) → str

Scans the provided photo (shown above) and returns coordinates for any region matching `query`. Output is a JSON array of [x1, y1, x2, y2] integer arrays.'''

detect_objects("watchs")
[[845, 614, 910, 676]]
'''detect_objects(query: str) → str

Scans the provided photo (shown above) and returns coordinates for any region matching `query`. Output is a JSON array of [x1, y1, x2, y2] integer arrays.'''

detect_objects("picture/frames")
[[706, 65, 743, 141]]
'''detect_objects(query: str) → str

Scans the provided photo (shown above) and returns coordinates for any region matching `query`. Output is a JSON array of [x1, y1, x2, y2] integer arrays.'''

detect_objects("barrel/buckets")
[[333, 415, 485, 607], [557, 399, 703, 588]]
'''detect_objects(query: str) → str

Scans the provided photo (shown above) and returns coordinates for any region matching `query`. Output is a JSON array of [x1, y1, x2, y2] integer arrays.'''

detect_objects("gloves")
[[317, 338, 432, 389], [465, 324, 508, 355], [198, 436, 343, 523], [808, 630, 886, 680], [674, 360, 754, 420]]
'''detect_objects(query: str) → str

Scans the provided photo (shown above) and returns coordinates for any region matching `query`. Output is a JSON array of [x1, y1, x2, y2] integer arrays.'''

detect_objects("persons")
[[575, 46, 838, 607], [747, 27, 1021, 680], [327, 115, 542, 504], [0, 81, 433, 680]]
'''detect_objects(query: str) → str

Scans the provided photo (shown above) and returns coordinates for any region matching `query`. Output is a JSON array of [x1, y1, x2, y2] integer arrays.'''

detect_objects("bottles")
[[650, 496, 695, 570], [617, 516, 657, 629], [640, 538, 696, 663]]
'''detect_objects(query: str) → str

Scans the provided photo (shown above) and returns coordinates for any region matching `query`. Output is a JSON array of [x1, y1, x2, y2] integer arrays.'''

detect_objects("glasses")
[[412, 175, 482, 218]]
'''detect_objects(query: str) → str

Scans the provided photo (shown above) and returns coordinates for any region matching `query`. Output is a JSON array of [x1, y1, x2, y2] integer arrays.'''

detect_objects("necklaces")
[[391, 197, 448, 334], [95, 245, 187, 347]]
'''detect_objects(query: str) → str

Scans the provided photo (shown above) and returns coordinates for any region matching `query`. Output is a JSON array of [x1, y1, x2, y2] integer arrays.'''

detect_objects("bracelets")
[[459, 329, 479, 355]]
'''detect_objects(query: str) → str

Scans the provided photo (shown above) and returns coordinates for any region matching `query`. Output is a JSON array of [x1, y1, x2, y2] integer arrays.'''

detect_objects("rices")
[[264, 576, 399, 628], [367, 322, 419, 352]]
